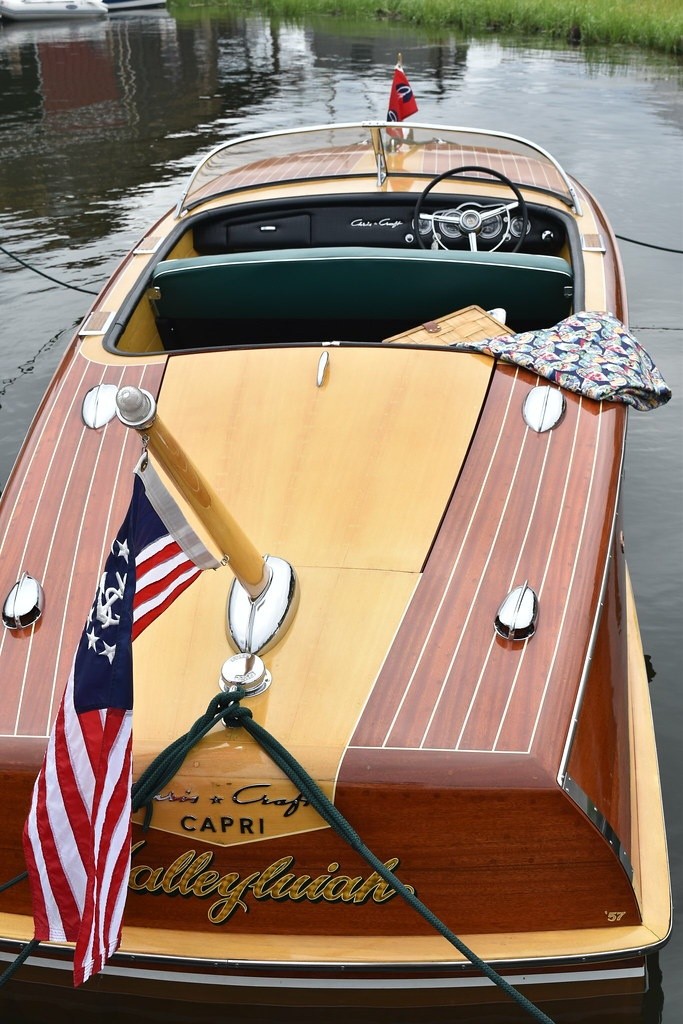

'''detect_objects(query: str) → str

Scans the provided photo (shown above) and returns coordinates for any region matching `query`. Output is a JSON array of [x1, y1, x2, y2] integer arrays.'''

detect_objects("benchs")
[[147, 245, 575, 349]]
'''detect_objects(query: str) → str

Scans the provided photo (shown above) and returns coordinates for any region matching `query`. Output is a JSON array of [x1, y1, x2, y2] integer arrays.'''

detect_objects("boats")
[[0, 112, 680, 1024]]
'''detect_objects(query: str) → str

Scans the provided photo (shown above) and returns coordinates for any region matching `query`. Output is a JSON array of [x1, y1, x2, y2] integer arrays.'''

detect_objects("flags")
[[21, 455, 220, 987], [385, 64, 419, 142]]
